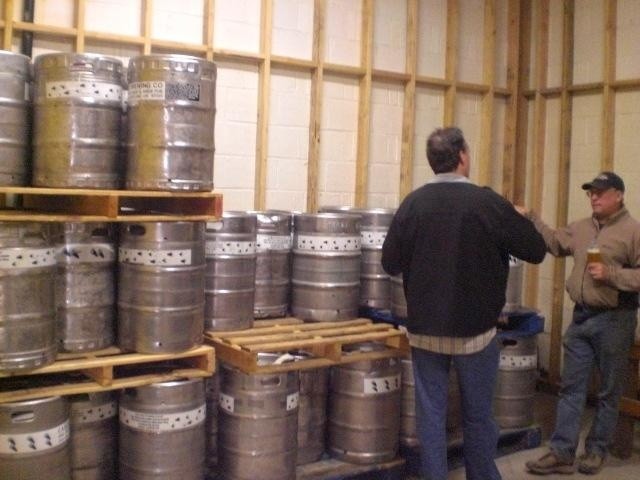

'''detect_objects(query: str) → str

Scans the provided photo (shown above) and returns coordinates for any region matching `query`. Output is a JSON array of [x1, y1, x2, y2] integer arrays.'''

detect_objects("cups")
[[586, 240, 602, 264]]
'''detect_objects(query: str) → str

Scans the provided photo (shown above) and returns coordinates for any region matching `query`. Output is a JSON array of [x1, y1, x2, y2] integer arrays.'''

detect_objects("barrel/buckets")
[[0, 207, 529, 371], [2, 53, 224, 184], [0, 330, 538, 479]]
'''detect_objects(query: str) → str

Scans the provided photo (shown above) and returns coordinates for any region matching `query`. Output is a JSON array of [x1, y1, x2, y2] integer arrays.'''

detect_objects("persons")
[[513, 172, 640, 475], [381, 127, 546, 480]]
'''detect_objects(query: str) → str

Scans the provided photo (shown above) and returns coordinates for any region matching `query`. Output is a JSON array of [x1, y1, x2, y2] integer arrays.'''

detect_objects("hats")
[[582, 172, 625, 192]]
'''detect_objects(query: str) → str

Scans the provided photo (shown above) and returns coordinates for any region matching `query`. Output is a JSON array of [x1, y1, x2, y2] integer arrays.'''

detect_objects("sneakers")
[[524, 452, 575, 474], [579, 453, 606, 474]]
